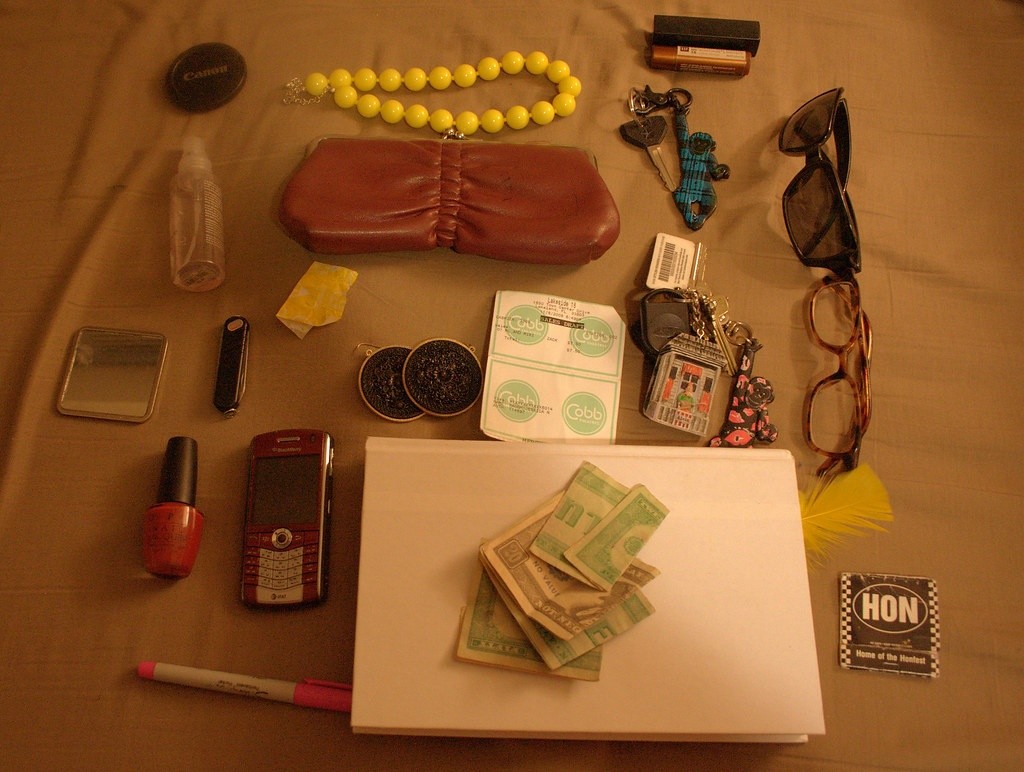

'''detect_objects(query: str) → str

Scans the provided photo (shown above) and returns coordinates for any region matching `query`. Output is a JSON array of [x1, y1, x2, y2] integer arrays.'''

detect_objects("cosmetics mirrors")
[[59, 326, 167, 423]]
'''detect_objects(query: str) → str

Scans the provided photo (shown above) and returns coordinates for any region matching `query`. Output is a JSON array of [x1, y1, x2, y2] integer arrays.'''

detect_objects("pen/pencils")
[[136, 659, 353, 715]]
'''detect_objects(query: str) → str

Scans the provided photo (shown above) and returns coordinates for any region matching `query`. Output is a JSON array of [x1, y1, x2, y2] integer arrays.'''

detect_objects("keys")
[[639, 240, 737, 375], [619, 114, 678, 192]]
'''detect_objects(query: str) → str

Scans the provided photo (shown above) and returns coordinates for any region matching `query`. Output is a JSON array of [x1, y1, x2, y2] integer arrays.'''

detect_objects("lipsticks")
[[651, 15, 760, 76]]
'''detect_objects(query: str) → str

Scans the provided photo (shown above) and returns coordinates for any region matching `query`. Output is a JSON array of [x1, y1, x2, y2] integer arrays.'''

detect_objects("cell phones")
[[241, 429, 334, 607]]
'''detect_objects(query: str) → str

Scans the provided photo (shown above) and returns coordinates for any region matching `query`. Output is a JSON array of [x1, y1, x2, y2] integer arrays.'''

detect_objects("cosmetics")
[[143, 434, 203, 577]]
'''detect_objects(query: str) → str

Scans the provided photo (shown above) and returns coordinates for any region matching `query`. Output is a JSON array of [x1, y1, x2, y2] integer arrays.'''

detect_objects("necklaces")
[[284, 51, 583, 135]]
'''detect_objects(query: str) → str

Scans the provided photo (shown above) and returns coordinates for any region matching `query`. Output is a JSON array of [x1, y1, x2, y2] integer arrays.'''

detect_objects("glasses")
[[802, 270, 874, 479], [778, 87, 861, 275]]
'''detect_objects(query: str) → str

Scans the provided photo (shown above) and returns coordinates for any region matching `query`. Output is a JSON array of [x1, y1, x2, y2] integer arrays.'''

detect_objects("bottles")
[[167, 136, 226, 294]]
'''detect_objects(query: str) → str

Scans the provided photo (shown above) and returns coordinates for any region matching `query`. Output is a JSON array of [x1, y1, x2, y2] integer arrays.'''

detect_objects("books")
[[349, 434, 828, 746]]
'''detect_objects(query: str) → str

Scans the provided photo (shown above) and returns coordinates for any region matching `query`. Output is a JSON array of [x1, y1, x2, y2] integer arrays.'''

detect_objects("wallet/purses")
[[276, 129, 619, 266]]
